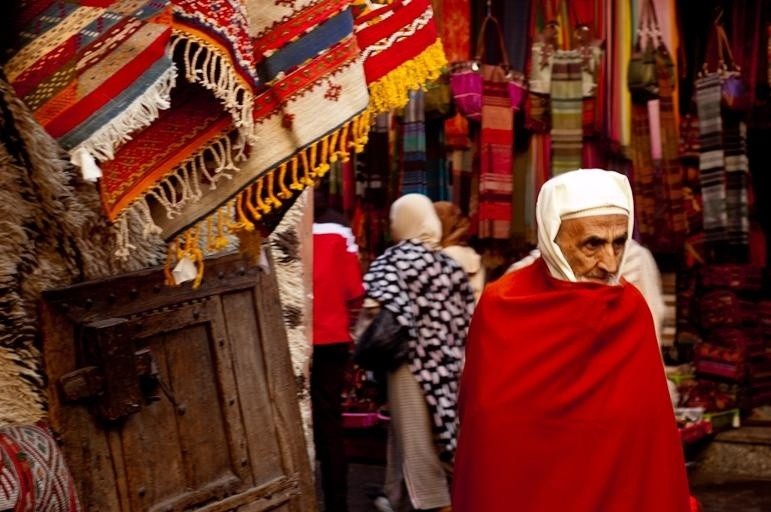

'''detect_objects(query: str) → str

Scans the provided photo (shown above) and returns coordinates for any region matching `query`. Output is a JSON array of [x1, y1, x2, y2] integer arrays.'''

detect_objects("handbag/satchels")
[[627, 32, 676, 101], [352, 296, 413, 375], [449, 54, 528, 126], [692, 50, 745, 117], [525, 29, 604, 99]]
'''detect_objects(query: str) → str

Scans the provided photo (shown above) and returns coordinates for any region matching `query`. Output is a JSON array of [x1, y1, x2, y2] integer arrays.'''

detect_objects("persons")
[[432, 200, 486, 304], [500, 230, 677, 406], [453, 168, 699, 511], [311, 198, 365, 511], [362, 193, 477, 512]]
